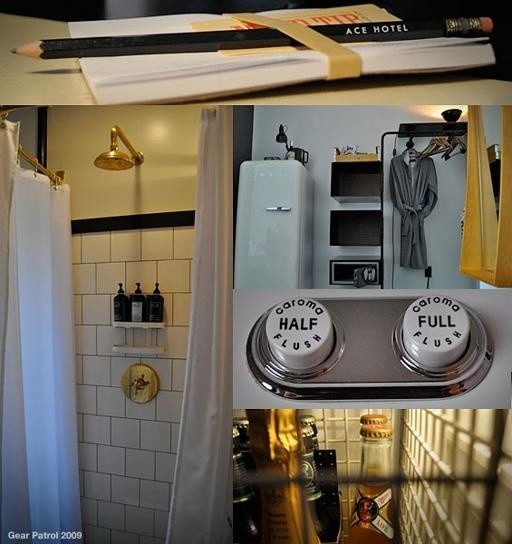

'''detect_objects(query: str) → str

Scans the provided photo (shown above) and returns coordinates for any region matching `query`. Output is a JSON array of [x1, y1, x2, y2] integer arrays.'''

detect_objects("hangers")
[[405, 134, 466, 162]]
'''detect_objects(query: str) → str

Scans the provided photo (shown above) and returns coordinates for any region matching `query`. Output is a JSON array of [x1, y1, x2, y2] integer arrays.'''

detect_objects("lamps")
[[441, 108, 462, 122], [277, 123, 293, 151]]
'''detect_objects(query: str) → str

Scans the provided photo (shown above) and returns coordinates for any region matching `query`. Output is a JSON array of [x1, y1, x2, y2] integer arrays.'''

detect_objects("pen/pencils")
[[10, 17, 492, 60]]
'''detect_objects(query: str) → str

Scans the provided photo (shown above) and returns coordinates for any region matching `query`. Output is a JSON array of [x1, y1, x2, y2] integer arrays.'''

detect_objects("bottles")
[[231, 415, 396, 543]]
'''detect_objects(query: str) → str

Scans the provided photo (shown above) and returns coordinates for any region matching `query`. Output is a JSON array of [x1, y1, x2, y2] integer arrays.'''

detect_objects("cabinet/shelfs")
[[110, 320, 165, 358], [327, 160, 383, 286]]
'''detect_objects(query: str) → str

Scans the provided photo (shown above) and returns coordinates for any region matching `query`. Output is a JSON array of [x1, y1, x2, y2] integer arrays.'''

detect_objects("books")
[[64, 3, 498, 104]]
